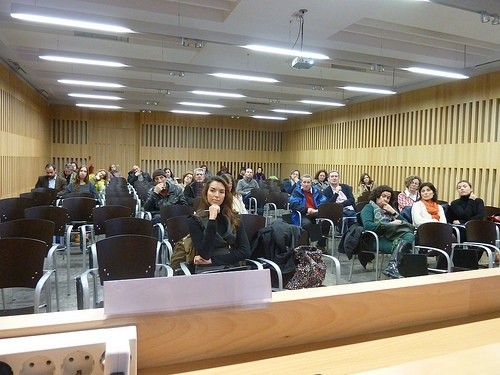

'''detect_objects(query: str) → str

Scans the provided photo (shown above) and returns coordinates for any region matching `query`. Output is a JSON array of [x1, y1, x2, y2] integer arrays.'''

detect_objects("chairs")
[[0, 175, 500, 316]]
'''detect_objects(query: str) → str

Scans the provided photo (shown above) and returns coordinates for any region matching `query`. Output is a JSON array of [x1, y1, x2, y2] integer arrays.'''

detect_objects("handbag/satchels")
[[285, 245, 327, 290]]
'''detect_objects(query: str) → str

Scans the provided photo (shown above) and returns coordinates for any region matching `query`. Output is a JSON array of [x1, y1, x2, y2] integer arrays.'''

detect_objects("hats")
[[153, 168, 167, 182]]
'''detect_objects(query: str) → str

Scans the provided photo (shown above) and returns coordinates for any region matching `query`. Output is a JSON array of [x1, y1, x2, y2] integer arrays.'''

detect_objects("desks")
[[0, 266, 500, 375]]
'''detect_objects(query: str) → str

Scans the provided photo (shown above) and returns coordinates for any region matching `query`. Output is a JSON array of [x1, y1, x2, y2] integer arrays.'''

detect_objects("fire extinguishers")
[[89, 163, 94, 174]]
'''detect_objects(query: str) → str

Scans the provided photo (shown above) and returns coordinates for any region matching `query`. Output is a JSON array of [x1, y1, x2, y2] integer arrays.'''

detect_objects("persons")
[[397, 175, 455, 270], [143, 168, 187, 223], [35, 164, 66, 197], [189, 176, 250, 273], [360, 185, 415, 278], [108, 164, 120, 182], [217, 166, 229, 175], [128, 165, 151, 185], [201, 165, 212, 176], [176, 172, 193, 191], [63, 165, 99, 241], [60, 164, 76, 184], [184, 168, 207, 206], [357, 173, 374, 196], [285, 169, 357, 255], [70, 162, 79, 173], [236, 167, 265, 209], [89, 169, 108, 191], [164, 168, 178, 184], [224, 174, 247, 214], [446, 181, 484, 242]]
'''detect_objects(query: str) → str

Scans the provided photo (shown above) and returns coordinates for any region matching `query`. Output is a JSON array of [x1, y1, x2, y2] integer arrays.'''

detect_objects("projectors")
[[291, 56, 315, 69]]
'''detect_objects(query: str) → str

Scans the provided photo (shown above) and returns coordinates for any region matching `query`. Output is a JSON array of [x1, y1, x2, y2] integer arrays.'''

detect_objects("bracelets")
[[392, 213, 396, 216]]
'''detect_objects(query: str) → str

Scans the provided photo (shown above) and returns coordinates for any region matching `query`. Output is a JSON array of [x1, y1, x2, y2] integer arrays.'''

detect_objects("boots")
[[382, 239, 411, 278]]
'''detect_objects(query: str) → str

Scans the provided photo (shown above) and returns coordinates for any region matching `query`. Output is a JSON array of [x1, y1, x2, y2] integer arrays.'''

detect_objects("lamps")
[[157, 89, 171, 95], [181, 37, 207, 48], [230, 115, 240, 119], [269, 99, 280, 104], [370, 63, 385, 72], [146, 100, 158, 105], [169, 71, 185, 77], [480, 10, 499, 25], [311, 84, 325, 91], [140, 109, 152, 113]]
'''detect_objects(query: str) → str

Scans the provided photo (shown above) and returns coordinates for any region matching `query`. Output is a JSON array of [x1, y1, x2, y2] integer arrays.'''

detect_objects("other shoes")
[[315, 243, 326, 253]]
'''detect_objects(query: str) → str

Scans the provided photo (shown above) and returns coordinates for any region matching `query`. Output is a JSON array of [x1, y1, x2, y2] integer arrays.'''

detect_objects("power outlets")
[[0, 326, 138, 375]]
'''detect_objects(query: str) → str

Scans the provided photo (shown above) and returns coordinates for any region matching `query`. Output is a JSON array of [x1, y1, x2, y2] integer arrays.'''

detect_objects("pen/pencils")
[[204, 210, 209, 211]]
